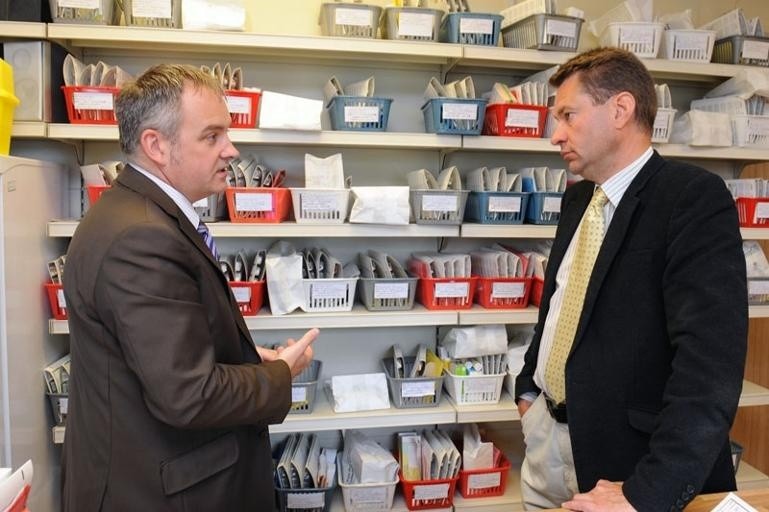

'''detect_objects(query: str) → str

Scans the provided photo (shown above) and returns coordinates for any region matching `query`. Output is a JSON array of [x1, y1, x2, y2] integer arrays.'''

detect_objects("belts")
[[539, 389, 569, 426]]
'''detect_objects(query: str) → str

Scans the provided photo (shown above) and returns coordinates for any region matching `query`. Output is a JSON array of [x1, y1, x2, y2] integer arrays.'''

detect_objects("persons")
[[63, 61, 320, 512], [513, 47, 750, 512]]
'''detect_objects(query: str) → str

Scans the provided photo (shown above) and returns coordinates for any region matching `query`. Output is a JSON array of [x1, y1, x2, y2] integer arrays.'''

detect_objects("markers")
[[462, 359, 483, 370]]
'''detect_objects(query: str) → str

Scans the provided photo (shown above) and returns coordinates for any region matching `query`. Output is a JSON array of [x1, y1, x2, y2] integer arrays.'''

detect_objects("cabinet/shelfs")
[[47, 22, 769, 512], [0, 21, 47, 139]]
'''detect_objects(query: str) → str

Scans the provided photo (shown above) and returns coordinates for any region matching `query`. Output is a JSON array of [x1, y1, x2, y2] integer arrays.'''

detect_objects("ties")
[[195, 215, 222, 270], [541, 185, 610, 404]]
